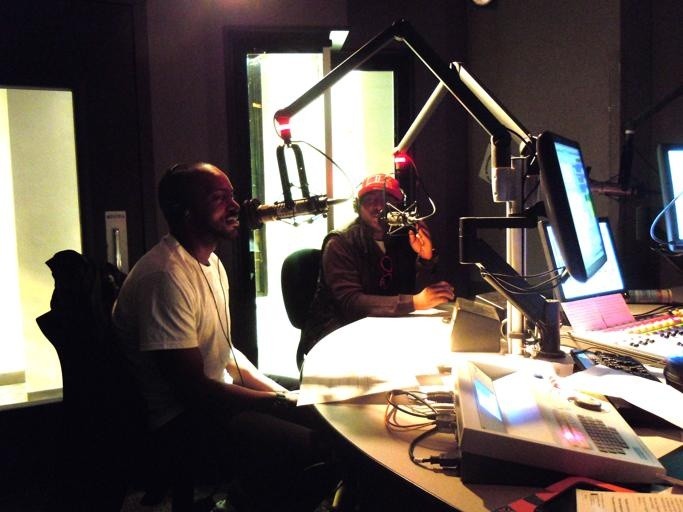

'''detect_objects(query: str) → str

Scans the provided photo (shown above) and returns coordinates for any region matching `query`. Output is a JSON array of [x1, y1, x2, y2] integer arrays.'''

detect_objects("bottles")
[[356, 173, 405, 205]]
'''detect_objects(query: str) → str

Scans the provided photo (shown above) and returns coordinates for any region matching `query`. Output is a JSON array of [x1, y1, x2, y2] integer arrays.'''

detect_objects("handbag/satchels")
[[270, 392, 290, 416]]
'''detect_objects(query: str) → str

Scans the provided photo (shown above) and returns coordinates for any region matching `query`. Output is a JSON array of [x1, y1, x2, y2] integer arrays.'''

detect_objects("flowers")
[[376, 211, 417, 229], [242, 195, 329, 231]]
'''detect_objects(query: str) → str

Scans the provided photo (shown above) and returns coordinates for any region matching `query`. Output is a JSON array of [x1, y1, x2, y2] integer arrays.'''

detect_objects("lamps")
[[279, 246, 321, 370], [46, 248, 204, 511]]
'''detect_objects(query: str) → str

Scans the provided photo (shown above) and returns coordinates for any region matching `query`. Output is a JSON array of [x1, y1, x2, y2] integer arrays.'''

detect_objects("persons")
[[295, 172, 456, 375], [103, 158, 343, 512]]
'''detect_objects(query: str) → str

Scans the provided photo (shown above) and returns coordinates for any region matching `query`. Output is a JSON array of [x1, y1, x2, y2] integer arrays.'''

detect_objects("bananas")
[[568, 348, 664, 412]]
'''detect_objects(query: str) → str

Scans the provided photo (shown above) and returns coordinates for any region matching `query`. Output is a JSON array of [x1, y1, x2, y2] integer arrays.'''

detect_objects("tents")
[[352, 174, 407, 213], [168, 160, 193, 233]]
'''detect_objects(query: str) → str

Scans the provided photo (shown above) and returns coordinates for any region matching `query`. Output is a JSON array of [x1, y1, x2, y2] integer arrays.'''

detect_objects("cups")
[[378, 253, 394, 290]]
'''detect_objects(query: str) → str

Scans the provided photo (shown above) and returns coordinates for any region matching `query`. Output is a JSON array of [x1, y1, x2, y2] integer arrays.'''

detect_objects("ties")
[[537, 220, 625, 302], [540, 131, 609, 284], [656, 144, 683, 253]]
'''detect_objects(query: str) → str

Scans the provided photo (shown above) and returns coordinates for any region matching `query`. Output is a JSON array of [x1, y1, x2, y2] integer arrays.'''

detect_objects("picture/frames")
[[298, 304, 680, 511]]
